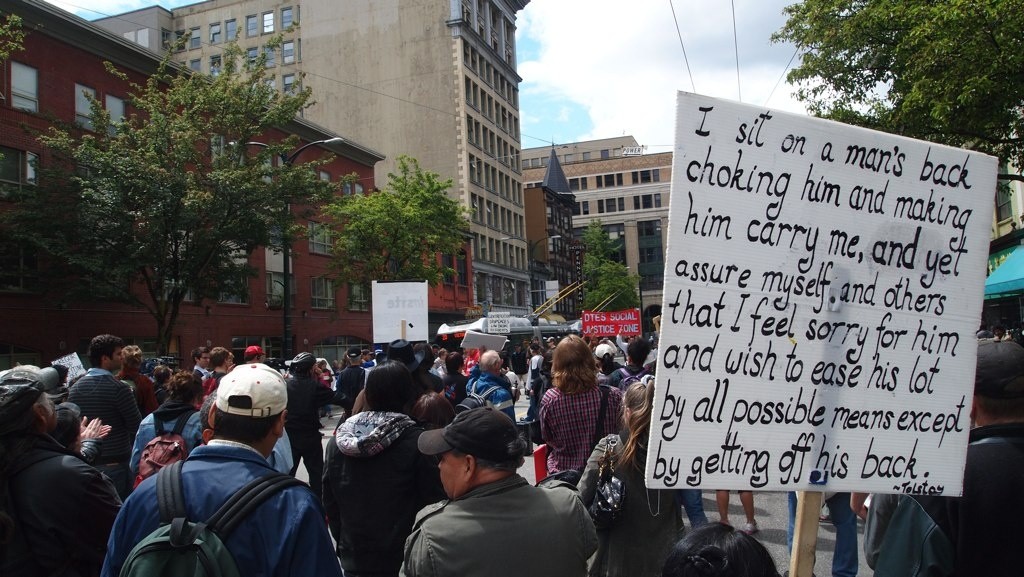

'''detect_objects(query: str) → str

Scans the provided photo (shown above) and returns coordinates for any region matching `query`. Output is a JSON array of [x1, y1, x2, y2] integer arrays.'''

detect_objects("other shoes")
[[520, 418, 525, 422]]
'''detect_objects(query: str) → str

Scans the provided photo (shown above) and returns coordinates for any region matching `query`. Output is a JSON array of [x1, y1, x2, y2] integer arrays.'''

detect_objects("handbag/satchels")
[[592, 434, 625, 529], [554, 470, 583, 486]]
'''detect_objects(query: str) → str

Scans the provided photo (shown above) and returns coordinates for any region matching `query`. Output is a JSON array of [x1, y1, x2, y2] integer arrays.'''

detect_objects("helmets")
[[291, 351, 316, 363]]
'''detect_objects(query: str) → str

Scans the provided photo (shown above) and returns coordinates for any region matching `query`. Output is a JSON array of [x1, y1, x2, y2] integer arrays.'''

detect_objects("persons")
[[662, 490, 905, 577], [100, 362, 344, 577], [576, 380, 679, 577], [874, 340, 1024, 577], [321, 360, 450, 577], [52, 330, 658, 504], [976, 319, 1024, 347], [398, 407, 600, 577], [0, 367, 125, 577]]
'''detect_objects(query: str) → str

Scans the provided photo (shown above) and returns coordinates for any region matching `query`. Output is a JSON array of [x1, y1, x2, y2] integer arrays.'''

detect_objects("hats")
[[216, 362, 288, 418], [50, 402, 81, 440], [244, 345, 266, 355], [347, 347, 362, 362], [595, 344, 614, 358], [376, 339, 425, 373], [362, 348, 374, 355], [417, 409, 518, 460], [0, 364, 59, 425]]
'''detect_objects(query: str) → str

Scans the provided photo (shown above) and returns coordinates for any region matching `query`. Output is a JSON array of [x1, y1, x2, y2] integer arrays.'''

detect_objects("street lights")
[[500, 235, 562, 313], [225, 135, 346, 362]]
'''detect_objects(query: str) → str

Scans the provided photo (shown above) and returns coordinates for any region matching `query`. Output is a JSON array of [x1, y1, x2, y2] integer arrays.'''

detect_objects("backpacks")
[[115, 373, 143, 401], [455, 381, 502, 414], [119, 460, 310, 577], [133, 409, 197, 490], [618, 368, 651, 390]]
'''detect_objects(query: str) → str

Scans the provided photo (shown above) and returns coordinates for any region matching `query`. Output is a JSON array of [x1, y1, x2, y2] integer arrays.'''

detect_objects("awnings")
[[983, 244, 1024, 294]]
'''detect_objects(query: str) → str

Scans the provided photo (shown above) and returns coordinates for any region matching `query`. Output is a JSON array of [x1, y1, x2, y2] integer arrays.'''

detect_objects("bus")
[[435, 274, 631, 360]]
